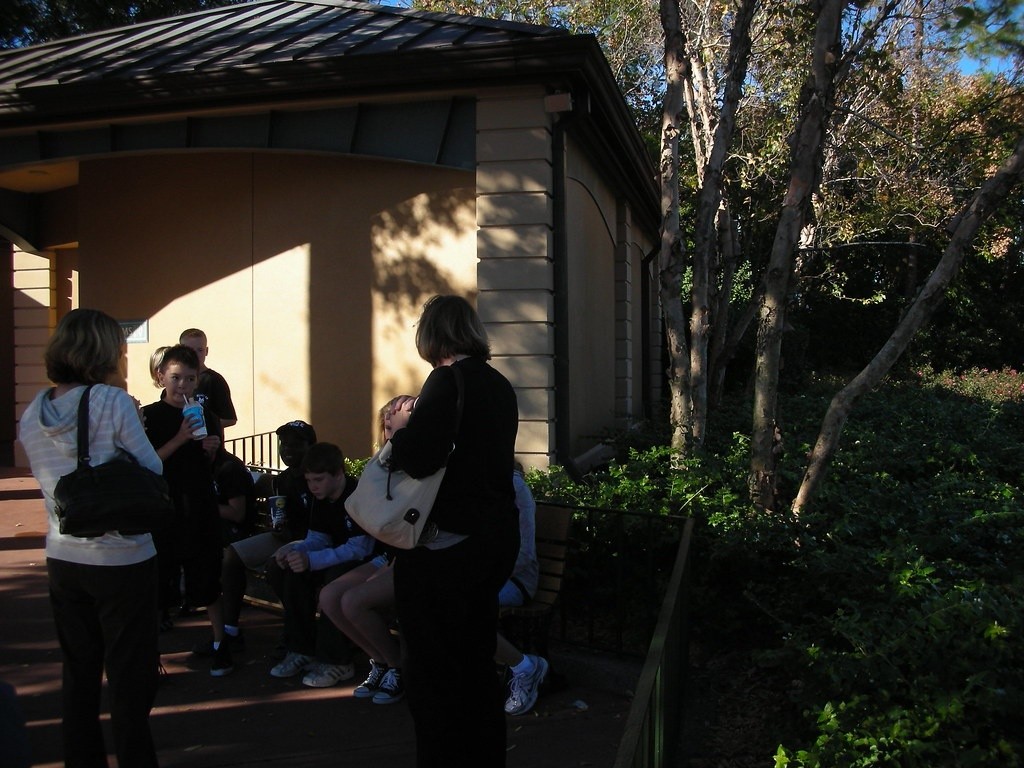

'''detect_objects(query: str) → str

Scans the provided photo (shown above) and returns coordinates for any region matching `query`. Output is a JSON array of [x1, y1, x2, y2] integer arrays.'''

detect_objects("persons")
[[139, 345, 236, 677], [161, 328, 237, 428], [270, 442, 375, 687], [18, 309, 165, 768], [211, 432, 257, 541], [193, 421, 317, 655], [0, 681, 31, 768], [390, 295, 520, 768], [496, 457, 549, 715], [319, 396, 417, 704]]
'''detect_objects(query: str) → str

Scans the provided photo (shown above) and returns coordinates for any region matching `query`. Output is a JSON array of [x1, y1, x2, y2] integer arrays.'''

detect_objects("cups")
[[268, 495, 288, 528], [182, 401, 208, 440]]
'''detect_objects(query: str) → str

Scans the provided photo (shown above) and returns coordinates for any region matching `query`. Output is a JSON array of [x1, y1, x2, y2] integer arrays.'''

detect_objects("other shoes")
[[192, 630, 245, 655]]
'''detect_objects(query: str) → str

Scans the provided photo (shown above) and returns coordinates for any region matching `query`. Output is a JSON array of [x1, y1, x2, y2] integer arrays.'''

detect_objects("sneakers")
[[303, 662, 354, 687], [354, 659, 406, 704], [210, 634, 233, 676], [271, 652, 318, 677], [505, 655, 548, 716]]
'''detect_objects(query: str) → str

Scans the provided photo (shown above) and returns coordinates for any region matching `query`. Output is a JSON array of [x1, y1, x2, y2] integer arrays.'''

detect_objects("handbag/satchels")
[[343, 366, 467, 549], [54, 384, 171, 537]]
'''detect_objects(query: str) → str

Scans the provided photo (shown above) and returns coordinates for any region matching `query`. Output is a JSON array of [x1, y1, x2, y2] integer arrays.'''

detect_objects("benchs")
[[230, 471, 573, 680]]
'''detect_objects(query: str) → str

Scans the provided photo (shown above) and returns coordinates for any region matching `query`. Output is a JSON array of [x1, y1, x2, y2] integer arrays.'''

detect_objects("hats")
[[276, 420, 316, 443]]
